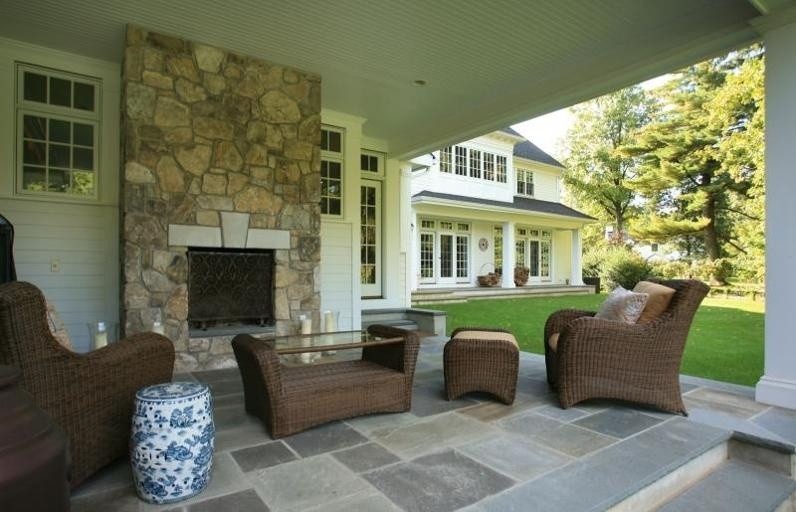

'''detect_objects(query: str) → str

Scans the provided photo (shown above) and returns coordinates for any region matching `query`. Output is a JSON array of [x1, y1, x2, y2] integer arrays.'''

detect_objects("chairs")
[[542, 275, 711, 418], [2, 280, 176, 494]]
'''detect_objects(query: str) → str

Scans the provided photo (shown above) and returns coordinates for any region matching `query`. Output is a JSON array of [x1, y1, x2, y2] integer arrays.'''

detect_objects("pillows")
[[44, 297, 73, 355], [593, 281, 676, 329]]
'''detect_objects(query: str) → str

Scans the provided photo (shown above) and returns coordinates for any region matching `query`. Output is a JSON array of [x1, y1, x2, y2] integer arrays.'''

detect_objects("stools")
[[442, 326, 520, 406], [130, 382, 216, 506]]
